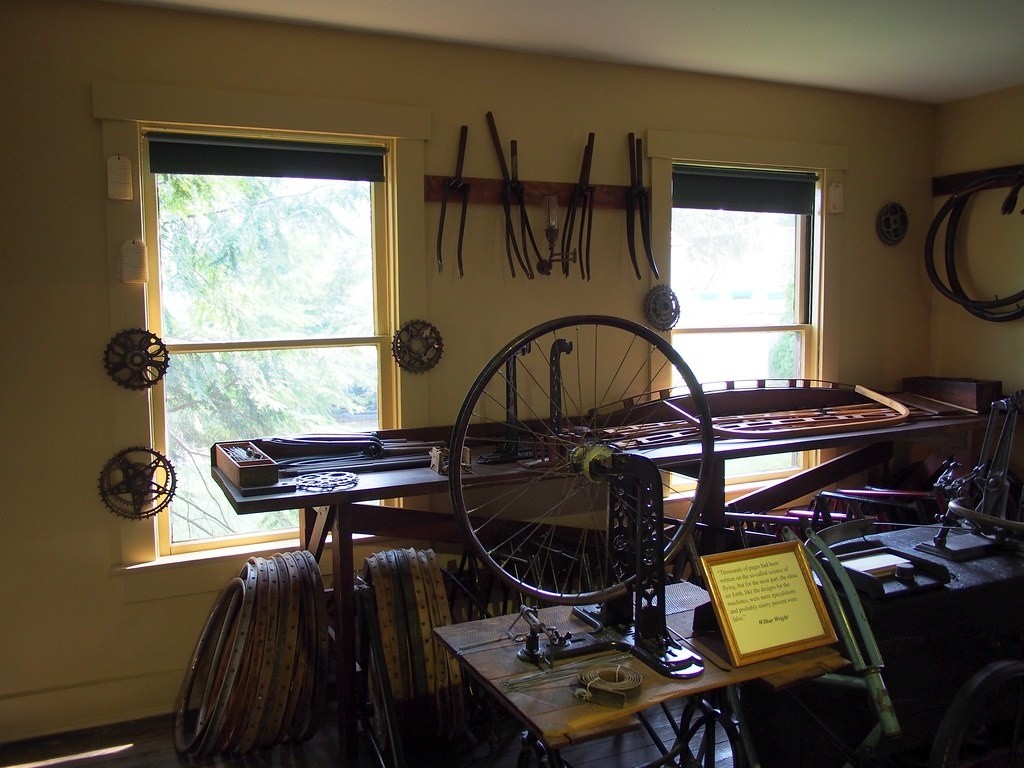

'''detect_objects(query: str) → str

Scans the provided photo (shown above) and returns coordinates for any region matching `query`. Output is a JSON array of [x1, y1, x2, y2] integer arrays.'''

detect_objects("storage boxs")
[[903, 376, 1002, 413]]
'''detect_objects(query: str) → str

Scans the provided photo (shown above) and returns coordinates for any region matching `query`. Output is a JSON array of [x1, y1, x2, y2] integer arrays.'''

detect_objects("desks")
[[211, 389, 1024, 767]]
